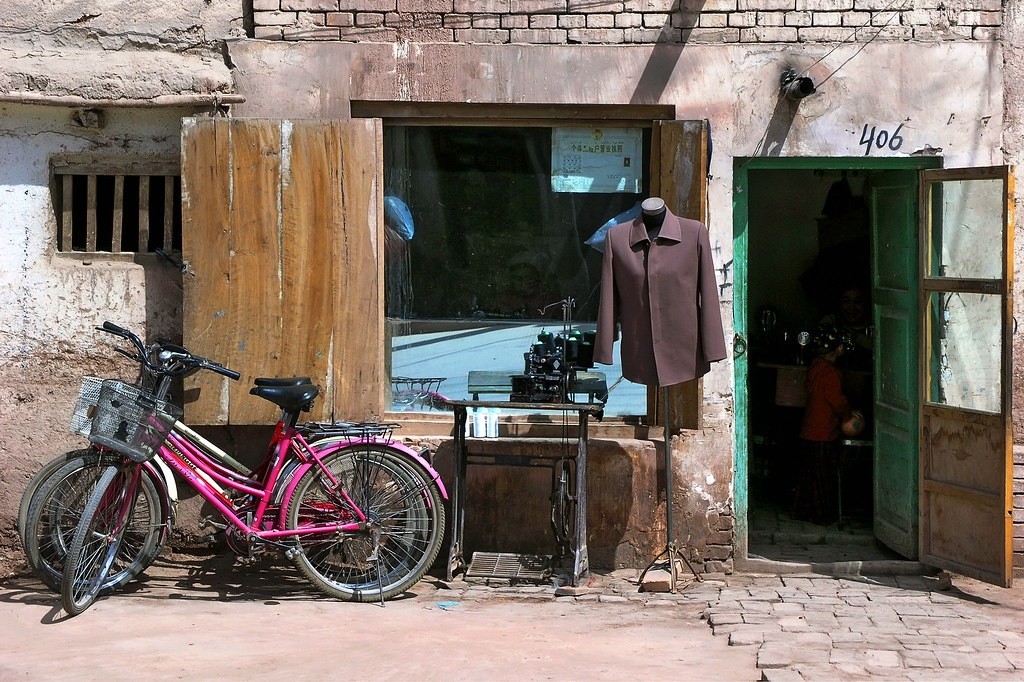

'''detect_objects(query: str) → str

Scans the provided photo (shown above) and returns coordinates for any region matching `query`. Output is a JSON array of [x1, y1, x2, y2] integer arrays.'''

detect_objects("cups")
[[487, 412, 499, 437], [473, 412, 485, 437]]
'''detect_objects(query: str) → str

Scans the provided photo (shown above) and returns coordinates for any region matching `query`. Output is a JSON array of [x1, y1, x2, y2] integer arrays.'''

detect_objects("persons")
[[787, 321, 864, 518], [593, 198, 727, 388]]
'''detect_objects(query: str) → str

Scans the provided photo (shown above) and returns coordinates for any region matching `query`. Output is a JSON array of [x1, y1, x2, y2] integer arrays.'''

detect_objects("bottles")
[[459, 398, 469, 437]]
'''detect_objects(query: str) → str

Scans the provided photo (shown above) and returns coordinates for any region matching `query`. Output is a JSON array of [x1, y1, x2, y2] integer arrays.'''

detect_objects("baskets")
[[68, 376, 164, 450], [88, 378, 183, 464]]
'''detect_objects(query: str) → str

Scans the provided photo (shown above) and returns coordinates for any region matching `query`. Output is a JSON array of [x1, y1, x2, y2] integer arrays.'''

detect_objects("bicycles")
[[18, 320, 448, 616]]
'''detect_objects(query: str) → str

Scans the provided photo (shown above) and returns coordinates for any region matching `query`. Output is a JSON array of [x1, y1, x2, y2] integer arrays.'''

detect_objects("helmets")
[[842, 415, 864, 436]]
